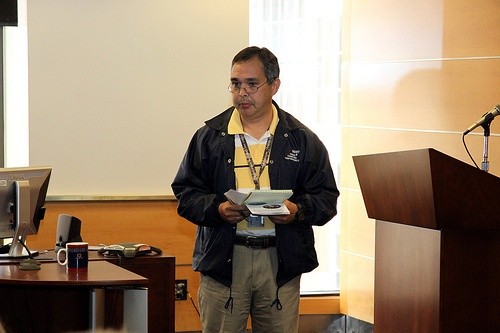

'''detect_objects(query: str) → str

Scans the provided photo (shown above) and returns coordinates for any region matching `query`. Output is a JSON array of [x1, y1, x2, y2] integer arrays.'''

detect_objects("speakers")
[[55, 214, 81, 253], [0, 0, 18, 26]]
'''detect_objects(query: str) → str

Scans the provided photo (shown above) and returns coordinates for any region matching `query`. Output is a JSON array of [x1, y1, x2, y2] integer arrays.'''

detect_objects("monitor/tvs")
[[0, 167, 52, 259]]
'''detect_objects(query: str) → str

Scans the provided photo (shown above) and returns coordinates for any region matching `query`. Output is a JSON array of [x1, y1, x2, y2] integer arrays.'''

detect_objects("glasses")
[[227, 76, 278, 94]]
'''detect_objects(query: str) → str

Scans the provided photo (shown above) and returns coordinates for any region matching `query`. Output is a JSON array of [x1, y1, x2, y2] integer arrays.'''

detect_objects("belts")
[[234, 235, 278, 250]]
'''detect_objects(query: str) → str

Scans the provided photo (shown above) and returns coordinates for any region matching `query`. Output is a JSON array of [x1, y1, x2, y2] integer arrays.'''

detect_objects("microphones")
[[463, 103, 500, 136]]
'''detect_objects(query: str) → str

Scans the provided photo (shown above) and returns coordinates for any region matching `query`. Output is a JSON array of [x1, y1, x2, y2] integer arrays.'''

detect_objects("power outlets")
[[175, 280, 187, 300]]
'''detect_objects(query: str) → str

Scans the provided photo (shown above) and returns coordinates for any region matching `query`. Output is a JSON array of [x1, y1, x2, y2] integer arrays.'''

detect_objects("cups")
[[56, 242, 89, 268]]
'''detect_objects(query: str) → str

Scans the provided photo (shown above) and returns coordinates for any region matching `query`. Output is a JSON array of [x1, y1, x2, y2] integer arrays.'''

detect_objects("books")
[[240, 189, 293, 216]]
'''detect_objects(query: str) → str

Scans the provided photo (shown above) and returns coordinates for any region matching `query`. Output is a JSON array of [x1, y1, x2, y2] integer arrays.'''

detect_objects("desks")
[[0, 250, 176, 333]]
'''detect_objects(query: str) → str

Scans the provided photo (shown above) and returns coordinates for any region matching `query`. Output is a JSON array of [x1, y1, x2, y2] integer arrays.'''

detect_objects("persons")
[[172, 46, 340, 333]]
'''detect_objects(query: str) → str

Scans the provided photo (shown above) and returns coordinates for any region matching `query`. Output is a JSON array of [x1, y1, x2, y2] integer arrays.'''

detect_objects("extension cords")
[[175, 280, 188, 301]]
